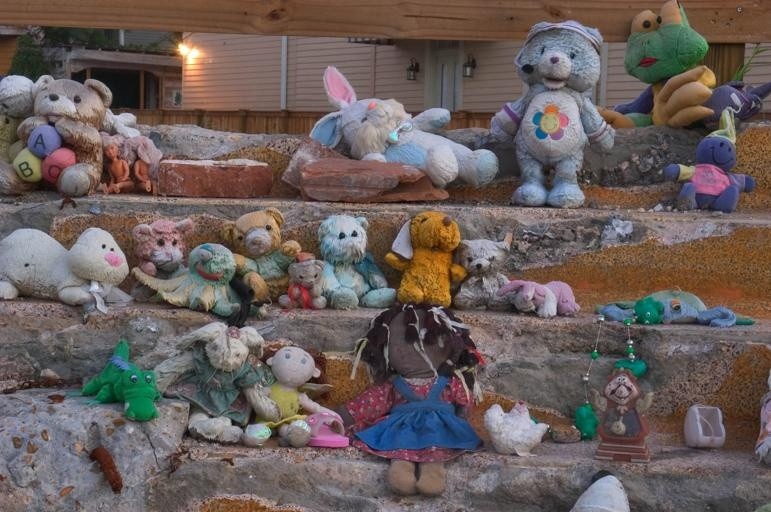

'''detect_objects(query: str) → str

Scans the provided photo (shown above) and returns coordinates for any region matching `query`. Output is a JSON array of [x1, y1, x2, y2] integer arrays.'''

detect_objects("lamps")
[[176, 32, 200, 59], [407, 56, 419, 82], [462, 52, 476, 78]]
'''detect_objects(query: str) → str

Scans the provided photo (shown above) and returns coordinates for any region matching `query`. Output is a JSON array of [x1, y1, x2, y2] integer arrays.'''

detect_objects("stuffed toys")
[[492, 1, 771, 213], [597, 288, 756, 329], [80, 306, 553, 498], [595, 372, 651, 438], [0, 203, 580, 317], [0, 0, 771, 496], [0, 76, 162, 197], [308, 65, 499, 190], [568, 470, 630, 512]]
